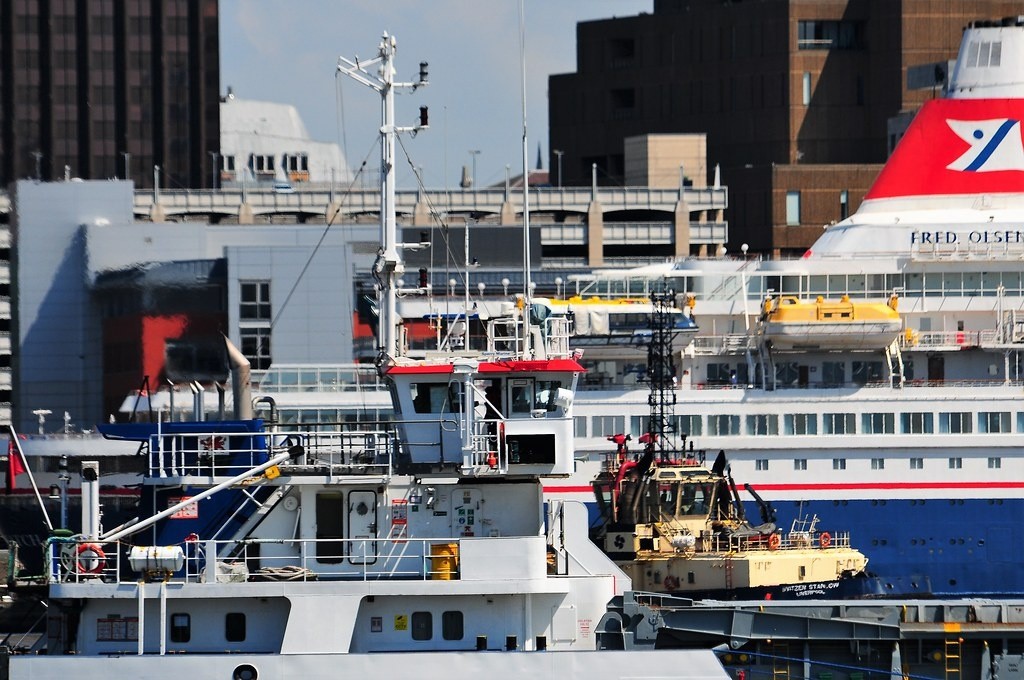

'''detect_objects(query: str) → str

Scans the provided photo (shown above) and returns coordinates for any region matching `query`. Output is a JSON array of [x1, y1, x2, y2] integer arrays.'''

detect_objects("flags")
[[5, 438, 24, 492]]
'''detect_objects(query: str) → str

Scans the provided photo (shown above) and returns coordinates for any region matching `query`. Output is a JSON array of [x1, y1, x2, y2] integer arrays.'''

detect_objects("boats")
[[0, 15, 1024, 679]]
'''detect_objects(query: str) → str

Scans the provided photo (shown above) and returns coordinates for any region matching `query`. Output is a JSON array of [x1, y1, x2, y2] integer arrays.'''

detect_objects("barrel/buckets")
[[431, 544, 458, 580]]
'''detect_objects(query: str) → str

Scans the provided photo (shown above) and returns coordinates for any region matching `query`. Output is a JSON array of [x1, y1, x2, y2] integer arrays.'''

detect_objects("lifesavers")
[[665, 576, 676, 591], [769, 533, 781, 549], [820, 532, 831, 547], [73, 543, 106, 573]]
